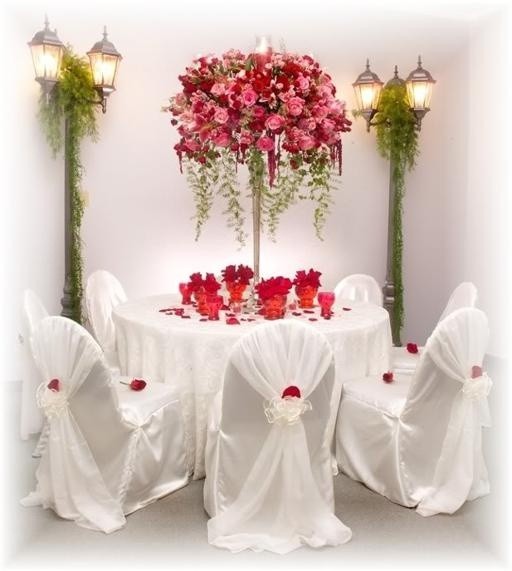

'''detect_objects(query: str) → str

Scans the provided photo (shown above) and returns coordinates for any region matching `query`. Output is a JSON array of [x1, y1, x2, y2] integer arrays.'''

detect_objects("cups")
[[179, 283, 192, 305], [206, 295, 223, 320], [318, 291, 335, 317]]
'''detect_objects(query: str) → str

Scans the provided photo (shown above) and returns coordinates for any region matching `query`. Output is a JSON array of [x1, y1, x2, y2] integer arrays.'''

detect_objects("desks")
[[112, 295, 391, 481]]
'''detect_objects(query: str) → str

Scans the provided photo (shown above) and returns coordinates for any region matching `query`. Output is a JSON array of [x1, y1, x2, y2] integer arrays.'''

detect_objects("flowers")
[[166, 42, 352, 243], [222, 264, 255, 302]]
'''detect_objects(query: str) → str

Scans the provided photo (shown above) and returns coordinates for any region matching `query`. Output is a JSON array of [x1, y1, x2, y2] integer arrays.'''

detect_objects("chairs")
[[336, 307, 491, 518], [335, 274, 384, 307], [392, 281, 477, 368], [85, 272, 129, 373], [29, 317, 190, 533], [204, 319, 352, 553]]
